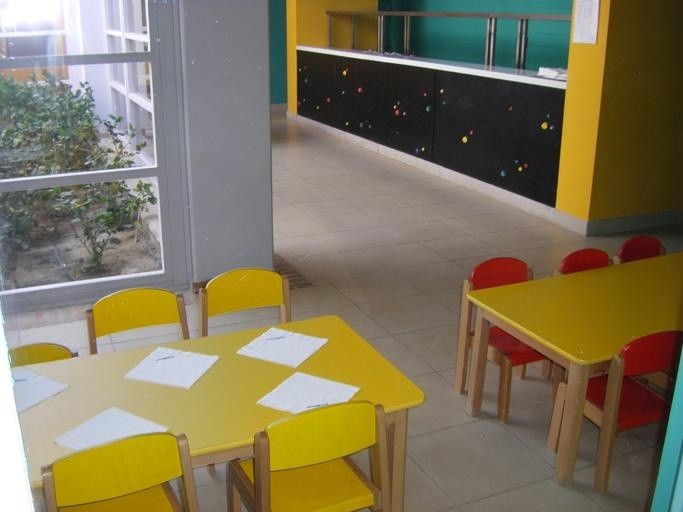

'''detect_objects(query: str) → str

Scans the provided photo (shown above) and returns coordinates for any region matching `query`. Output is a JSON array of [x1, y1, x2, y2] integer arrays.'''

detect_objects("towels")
[[256, 372, 362, 414], [124, 346, 221, 390], [236, 327, 329, 368], [10, 367, 69, 414], [52, 406, 172, 452]]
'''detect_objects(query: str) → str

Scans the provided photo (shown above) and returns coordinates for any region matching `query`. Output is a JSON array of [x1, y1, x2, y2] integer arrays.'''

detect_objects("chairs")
[[613, 235, 666, 264], [41, 432, 199, 512], [546, 330, 683, 496], [226, 400, 391, 512], [8, 342, 78, 369], [84, 287, 190, 355], [199, 267, 291, 337], [455, 257, 564, 424], [542, 248, 614, 379]]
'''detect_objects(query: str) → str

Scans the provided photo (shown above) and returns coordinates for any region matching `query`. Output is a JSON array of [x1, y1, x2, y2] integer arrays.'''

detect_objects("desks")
[[465, 251, 683, 487], [11, 314, 426, 512]]
